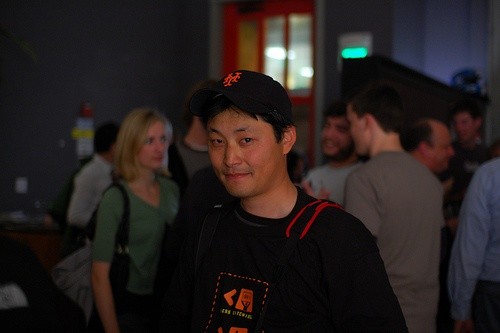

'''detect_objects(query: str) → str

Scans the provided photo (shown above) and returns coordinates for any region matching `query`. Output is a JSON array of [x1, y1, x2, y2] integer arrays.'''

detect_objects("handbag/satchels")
[[61, 183, 132, 299]]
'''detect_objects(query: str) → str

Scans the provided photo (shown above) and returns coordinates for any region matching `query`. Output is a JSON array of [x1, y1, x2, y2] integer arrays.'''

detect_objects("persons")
[[287, 81, 500, 333], [188, 70, 408, 333], [49, 80, 242, 333]]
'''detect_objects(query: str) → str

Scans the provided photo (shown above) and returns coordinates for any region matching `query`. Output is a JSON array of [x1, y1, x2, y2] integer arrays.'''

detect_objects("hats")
[[186, 68, 294, 123]]
